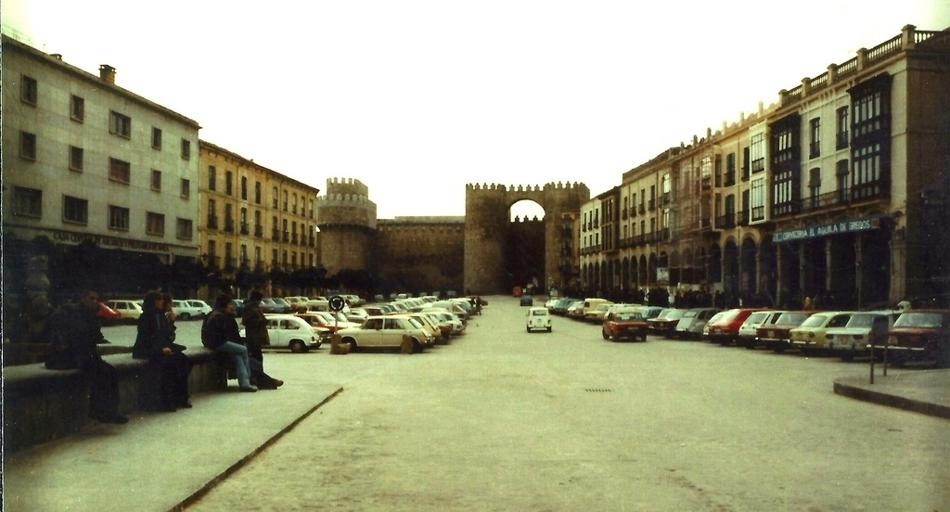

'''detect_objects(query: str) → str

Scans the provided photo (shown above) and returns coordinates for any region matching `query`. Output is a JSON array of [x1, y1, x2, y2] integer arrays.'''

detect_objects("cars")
[[512, 282, 583, 318], [524, 306, 552, 333]]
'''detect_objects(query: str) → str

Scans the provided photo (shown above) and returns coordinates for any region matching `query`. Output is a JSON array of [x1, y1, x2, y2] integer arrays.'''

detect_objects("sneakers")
[[86, 409, 134, 425], [239, 378, 284, 392], [156, 399, 192, 412]]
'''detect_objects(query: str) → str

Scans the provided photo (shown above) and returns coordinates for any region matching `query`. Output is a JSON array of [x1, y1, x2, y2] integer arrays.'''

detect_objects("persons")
[[132, 288, 282, 412], [45, 290, 129, 423]]
